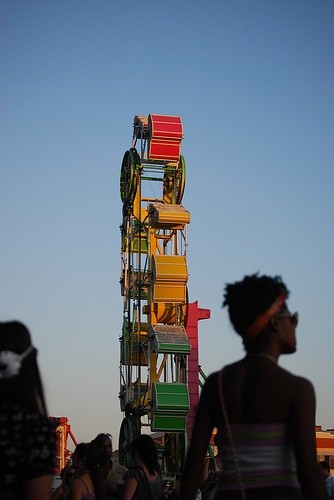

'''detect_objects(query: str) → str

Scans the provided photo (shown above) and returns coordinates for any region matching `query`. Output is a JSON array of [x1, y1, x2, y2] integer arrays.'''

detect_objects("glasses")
[[275, 312, 298, 327]]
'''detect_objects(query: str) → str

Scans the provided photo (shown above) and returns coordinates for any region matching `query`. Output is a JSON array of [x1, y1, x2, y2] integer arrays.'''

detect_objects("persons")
[[0, 321, 56, 500], [123, 434, 163, 500], [52, 433, 129, 500], [319, 461, 334, 500], [199, 433, 222, 500], [176, 271, 330, 500]]
